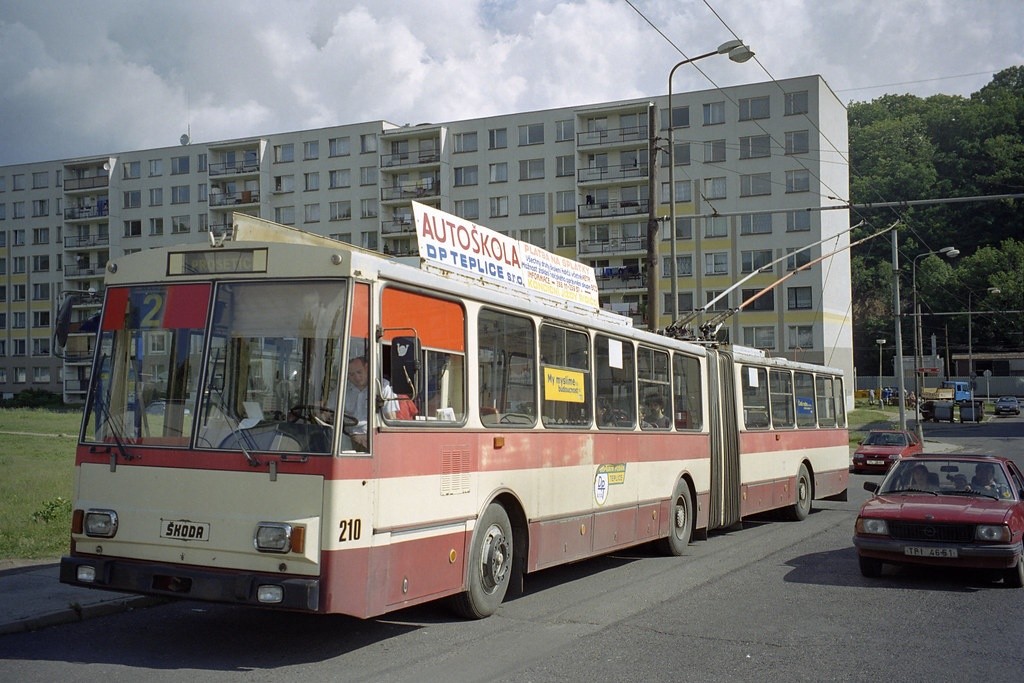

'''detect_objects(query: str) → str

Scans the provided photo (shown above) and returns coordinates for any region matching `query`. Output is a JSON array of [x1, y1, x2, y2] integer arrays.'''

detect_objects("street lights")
[[909, 247, 967, 444], [665, 37, 756, 334], [961, 286, 1001, 430]]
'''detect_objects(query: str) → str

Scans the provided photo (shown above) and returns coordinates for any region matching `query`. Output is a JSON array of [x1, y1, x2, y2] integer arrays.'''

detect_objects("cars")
[[852, 429, 923, 475], [994, 396, 1020, 417], [852, 453, 1024, 589]]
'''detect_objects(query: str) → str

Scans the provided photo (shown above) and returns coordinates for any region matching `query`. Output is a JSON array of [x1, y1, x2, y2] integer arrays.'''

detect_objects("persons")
[[637, 403, 654, 429], [869, 388, 875, 404], [908, 391, 916, 411], [344, 357, 401, 439], [643, 393, 672, 428], [594, 397, 619, 427], [882, 389, 891, 404], [908, 465, 943, 495], [952, 474, 968, 491], [963, 463, 1009, 499]]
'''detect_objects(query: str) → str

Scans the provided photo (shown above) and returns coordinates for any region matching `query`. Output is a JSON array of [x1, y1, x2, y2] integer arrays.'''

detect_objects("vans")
[[941, 380, 973, 403]]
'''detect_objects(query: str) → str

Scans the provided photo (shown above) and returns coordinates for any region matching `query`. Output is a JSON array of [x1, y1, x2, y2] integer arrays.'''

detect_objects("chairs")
[[906, 473, 939, 488], [486, 415, 649, 427], [971, 475, 998, 493]]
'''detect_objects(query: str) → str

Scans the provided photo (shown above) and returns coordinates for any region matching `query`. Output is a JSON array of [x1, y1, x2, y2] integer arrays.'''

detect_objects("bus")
[[59, 201, 853, 632]]
